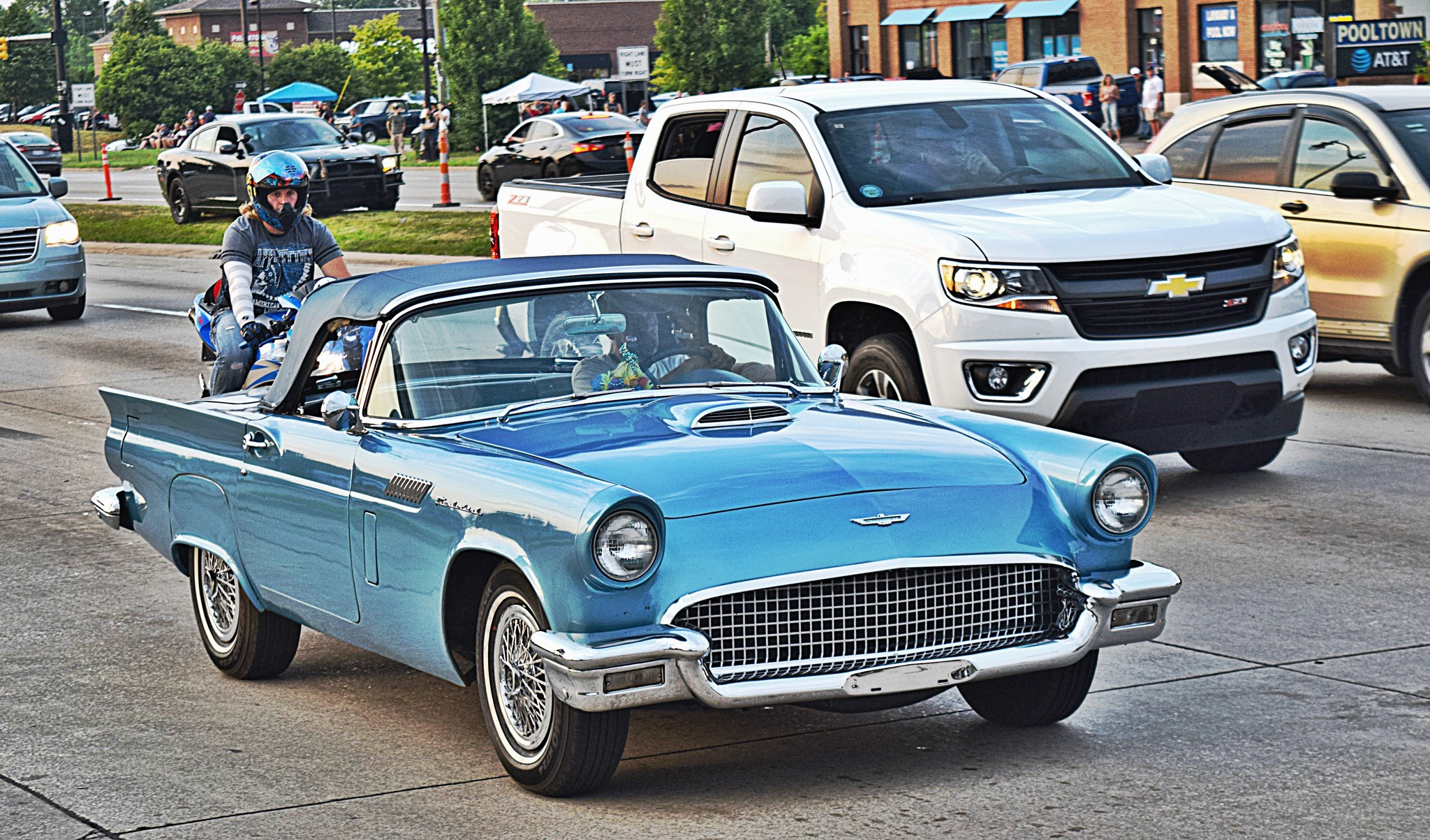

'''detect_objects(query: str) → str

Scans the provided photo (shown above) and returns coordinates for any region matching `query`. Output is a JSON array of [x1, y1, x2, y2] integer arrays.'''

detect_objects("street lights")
[[249, 1, 265, 97], [83, 9, 92, 82]]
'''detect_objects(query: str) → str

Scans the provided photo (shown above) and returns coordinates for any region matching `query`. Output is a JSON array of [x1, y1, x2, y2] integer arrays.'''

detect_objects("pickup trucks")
[[489, 78, 1323, 473], [992, 56, 1140, 137]]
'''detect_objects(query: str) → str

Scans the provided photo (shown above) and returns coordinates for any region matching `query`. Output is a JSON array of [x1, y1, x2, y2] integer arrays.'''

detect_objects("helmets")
[[246, 149, 310, 231]]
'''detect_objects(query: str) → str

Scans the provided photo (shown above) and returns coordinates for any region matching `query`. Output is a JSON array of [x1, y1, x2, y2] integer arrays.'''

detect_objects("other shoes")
[[1116, 139, 1120, 144], [1138, 136, 1149, 140], [1146, 138, 1155, 142]]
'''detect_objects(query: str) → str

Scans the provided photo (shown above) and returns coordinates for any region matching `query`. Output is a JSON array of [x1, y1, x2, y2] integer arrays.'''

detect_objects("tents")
[[256, 82, 338, 112], [482, 72, 593, 152]]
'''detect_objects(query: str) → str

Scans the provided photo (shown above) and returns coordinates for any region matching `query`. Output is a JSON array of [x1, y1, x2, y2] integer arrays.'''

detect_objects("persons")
[[418, 101, 451, 163], [707, 123, 722, 131], [1130, 67, 1152, 140], [697, 89, 705, 95], [678, 127, 690, 147], [209, 150, 352, 396], [1099, 74, 1121, 144], [1142, 65, 1163, 142], [896, 126, 1014, 192], [638, 100, 648, 127], [604, 92, 622, 114], [990, 70, 1003, 81], [315, 101, 334, 126], [519, 95, 575, 121], [68, 102, 100, 131], [571, 300, 777, 393], [348, 109, 361, 144], [673, 90, 682, 100], [140, 105, 216, 150], [823, 66, 870, 83], [386, 106, 407, 161], [505, 135, 526, 143]]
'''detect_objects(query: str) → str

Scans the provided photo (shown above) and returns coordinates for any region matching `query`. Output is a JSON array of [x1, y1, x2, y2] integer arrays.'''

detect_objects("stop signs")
[[235, 91, 246, 111]]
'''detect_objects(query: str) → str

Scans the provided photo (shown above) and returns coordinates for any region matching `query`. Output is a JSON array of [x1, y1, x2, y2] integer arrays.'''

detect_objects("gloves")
[[242, 322, 275, 349]]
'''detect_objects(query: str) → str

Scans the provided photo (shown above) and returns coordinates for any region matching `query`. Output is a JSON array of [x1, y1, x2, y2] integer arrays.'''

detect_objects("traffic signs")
[[234, 81, 248, 89]]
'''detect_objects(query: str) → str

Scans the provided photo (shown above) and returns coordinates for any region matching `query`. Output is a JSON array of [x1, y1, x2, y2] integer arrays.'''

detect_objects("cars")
[[0, 136, 87, 321], [1132, 85, 1430, 399], [629, 92, 691, 130], [1, 131, 63, 176], [1197, 64, 1326, 94], [156, 112, 407, 226], [0, 101, 63, 128], [69, 104, 122, 130], [335, 89, 439, 141], [476, 111, 650, 201], [99, 255, 1185, 799]]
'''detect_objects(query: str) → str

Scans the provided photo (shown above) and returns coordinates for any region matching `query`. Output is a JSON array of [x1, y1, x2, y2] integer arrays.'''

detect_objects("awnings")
[[932, 4, 1005, 22], [879, 9, 937, 25], [1003, 0, 1078, 18]]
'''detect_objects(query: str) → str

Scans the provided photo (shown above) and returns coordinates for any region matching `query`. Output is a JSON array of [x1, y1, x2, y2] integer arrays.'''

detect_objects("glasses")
[[639, 325, 659, 335]]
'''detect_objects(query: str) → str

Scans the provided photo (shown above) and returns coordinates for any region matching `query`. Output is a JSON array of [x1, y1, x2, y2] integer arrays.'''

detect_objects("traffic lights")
[[0, 37, 9, 60]]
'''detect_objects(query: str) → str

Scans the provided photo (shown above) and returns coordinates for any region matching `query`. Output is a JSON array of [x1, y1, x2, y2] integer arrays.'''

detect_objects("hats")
[[1130, 67, 1140, 75], [1145, 66, 1153, 71], [394, 106, 399, 110], [160, 124, 166, 129], [206, 106, 213, 112], [184, 109, 188, 113], [322, 102, 329, 107]]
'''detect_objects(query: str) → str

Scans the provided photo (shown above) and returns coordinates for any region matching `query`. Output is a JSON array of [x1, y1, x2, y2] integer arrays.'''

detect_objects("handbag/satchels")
[[448, 123, 456, 132]]
